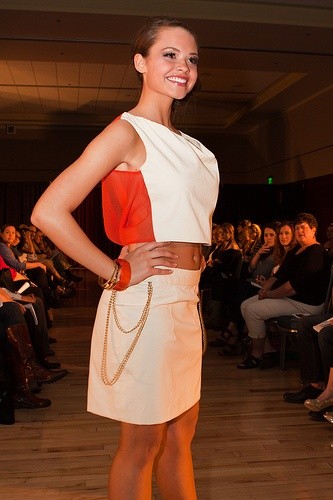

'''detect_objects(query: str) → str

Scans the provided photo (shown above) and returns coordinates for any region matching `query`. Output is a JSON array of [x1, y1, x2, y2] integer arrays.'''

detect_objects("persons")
[[0, 224, 83, 425], [30, 18, 221, 500], [202, 212, 333, 424]]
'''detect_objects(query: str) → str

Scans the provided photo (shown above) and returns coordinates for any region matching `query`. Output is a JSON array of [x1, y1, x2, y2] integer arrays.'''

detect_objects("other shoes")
[[304, 394, 332, 423], [238, 352, 279, 371], [8, 322, 69, 394], [14, 395, 52, 410], [284, 384, 322, 404]]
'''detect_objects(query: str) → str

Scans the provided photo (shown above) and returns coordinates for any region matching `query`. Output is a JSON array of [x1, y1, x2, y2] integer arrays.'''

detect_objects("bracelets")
[[99, 259, 131, 292]]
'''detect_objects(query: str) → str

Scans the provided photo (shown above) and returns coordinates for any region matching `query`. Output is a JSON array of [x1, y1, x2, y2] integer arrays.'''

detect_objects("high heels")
[[208, 329, 246, 356]]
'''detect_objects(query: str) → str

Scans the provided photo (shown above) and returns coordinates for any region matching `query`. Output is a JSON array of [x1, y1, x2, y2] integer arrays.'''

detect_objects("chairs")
[[266, 322, 298, 373]]
